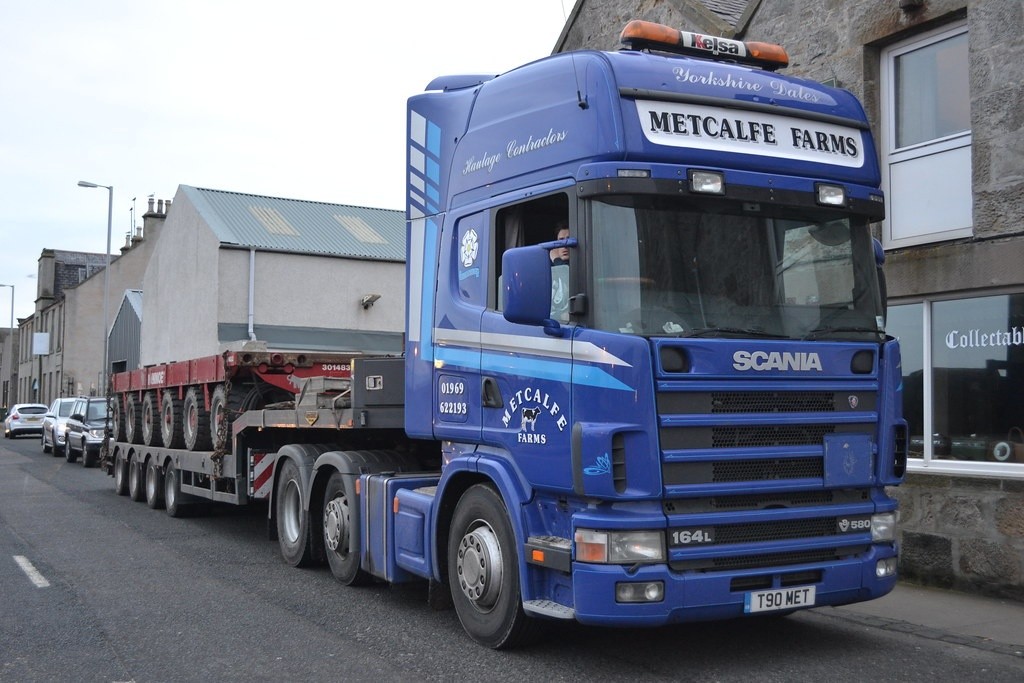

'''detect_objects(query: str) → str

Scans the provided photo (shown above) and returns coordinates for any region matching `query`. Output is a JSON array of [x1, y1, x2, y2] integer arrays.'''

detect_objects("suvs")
[[41, 396, 115, 467], [5, 403, 50, 438]]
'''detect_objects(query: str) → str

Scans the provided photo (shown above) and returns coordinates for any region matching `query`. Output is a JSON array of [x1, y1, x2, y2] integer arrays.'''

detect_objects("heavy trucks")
[[107, 21, 908, 652]]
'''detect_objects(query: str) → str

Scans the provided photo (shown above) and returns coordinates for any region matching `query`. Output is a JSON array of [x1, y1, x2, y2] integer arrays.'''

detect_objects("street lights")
[[0, 284, 14, 414], [77, 180, 113, 395]]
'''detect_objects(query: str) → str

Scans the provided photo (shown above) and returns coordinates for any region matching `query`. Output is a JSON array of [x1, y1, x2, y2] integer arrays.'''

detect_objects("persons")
[[549, 223, 581, 265]]
